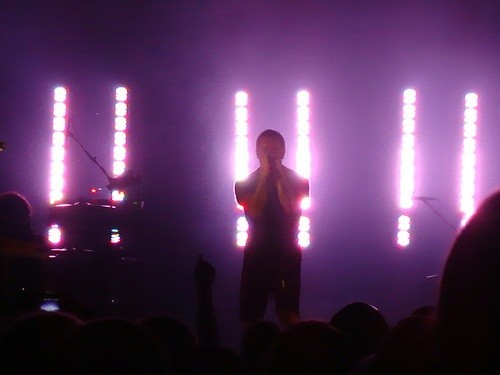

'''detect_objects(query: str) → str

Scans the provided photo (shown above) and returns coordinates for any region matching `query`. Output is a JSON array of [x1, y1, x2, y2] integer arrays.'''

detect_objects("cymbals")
[[50, 196, 112, 208]]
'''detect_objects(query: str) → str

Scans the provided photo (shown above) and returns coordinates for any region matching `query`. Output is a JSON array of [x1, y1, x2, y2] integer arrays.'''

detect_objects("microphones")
[[267, 152, 279, 178], [414, 195, 437, 202]]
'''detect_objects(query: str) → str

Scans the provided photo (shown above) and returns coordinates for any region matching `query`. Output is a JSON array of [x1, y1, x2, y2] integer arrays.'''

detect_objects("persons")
[[0, 287, 500, 372], [232, 129, 310, 334], [433, 188, 500, 372]]
